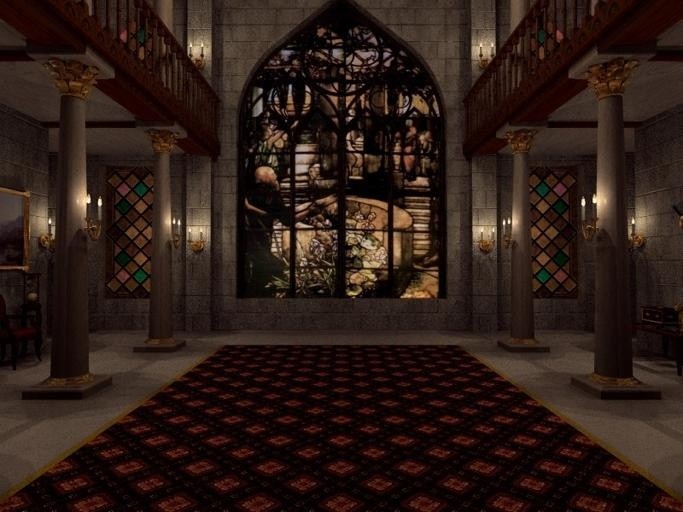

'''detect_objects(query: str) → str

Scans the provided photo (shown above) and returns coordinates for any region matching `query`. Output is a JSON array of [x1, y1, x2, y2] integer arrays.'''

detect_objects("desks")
[[633, 321, 683, 377]]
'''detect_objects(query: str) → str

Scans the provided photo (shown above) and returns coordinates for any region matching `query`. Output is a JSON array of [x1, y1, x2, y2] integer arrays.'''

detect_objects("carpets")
[[1, 344, 681, 510]]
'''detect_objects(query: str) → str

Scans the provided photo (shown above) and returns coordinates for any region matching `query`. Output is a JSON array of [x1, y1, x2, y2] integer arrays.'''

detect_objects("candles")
[[502, 217, 511, 236], [632, 216, 635, 235], [47, 217, 52, 233], [172, 217, 181, 235], [189, 41, 204, 55], [87, 193, 103, 221], [479, 42, 494, 56], [480, 227, 495, 241], [188, 227, 202, 240], [580, 195, 598, 222]]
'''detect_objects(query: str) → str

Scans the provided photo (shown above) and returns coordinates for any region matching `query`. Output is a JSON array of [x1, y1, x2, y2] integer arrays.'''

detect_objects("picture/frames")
[[0, 186, 32, 276]]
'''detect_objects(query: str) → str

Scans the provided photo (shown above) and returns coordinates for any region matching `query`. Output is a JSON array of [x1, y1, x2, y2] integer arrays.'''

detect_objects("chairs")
[[0, 294, 43, 371]]
